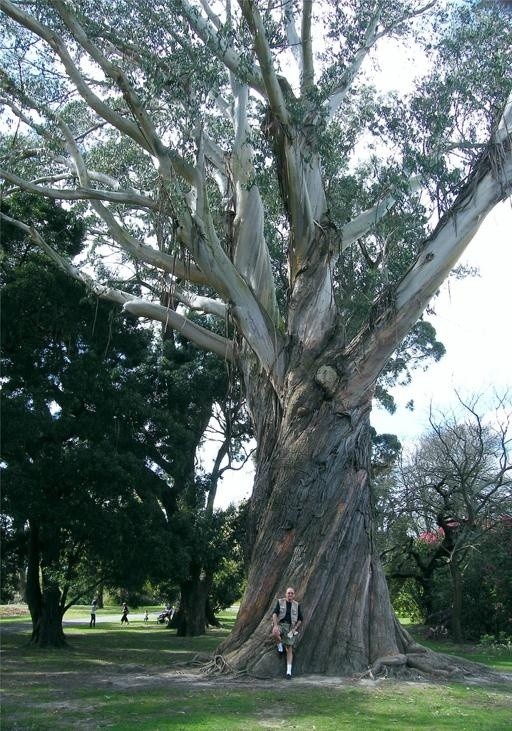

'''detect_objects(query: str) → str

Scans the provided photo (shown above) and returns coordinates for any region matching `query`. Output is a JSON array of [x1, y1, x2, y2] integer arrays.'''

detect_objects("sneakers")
[[277, 643, 283, 652], [287, 673, 291, 676]]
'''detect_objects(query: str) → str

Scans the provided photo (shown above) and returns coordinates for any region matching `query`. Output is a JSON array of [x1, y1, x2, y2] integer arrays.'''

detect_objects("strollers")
[[156, 611, 170, 625]]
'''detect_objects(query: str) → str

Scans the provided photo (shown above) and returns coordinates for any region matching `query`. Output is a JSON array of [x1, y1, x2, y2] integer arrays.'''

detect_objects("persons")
[[143, 609, 148, 623], [272, 587, 303, 679], [119, 601, 129, 625], [89, 600, 98, 627]]
